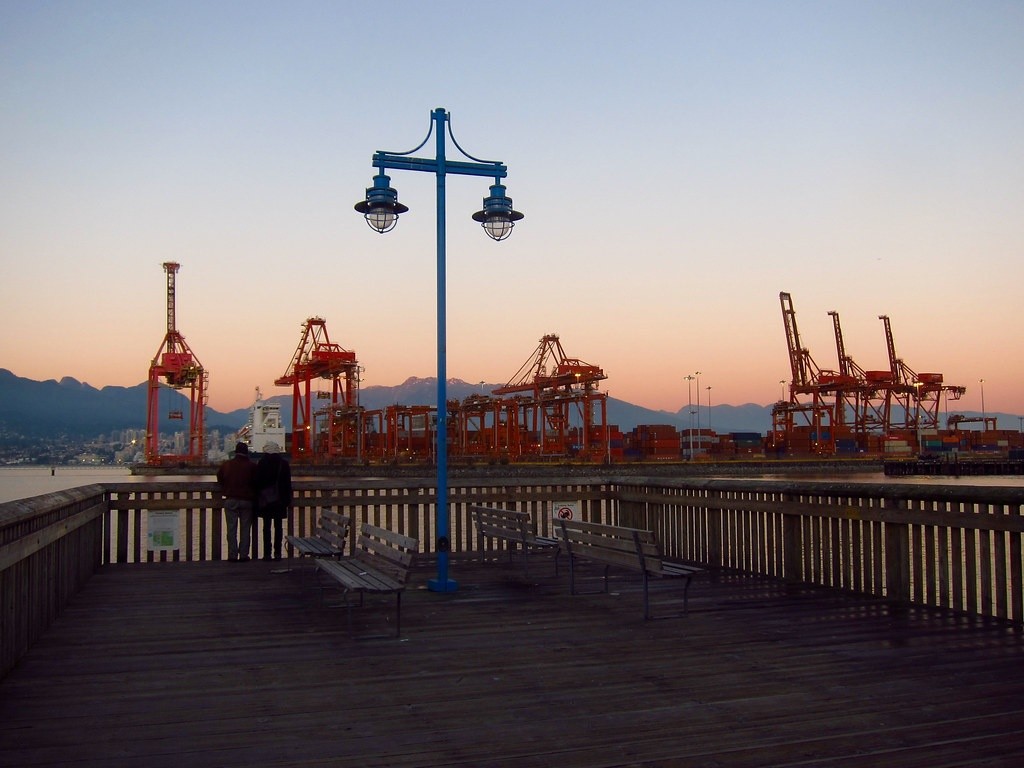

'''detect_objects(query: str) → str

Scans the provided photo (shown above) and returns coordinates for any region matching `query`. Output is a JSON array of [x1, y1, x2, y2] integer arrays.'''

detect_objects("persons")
[[216, 442, 294, 563]]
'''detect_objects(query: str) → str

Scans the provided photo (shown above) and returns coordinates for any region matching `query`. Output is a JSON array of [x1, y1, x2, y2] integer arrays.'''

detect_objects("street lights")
[[355, 102, 526, 594], [683, 371, 714, 460], [977, 378, 986, 430], [779, 380, 786, 401]]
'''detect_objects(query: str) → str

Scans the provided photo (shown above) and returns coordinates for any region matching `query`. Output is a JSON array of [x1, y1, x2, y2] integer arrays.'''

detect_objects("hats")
[[264, 441, 280, 453], [236, 442, 248, 455]]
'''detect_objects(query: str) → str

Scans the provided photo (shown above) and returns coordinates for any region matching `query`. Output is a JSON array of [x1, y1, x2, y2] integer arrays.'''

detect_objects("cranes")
[[144, 261, 209, 464], [765, 290, 967, 455], [275, 315, 614, 465]]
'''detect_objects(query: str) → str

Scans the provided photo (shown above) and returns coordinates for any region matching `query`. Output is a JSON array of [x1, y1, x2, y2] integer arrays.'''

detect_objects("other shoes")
[[239, 556, 250, 561], [228, 559, 238, 562]]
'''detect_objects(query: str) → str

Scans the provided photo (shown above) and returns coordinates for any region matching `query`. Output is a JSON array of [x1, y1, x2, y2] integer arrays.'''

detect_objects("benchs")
[[271, 509, 352, 588], [552, 517, 706, 621], [319, 524, 420, 641], [470, 505, 562, 578]]
